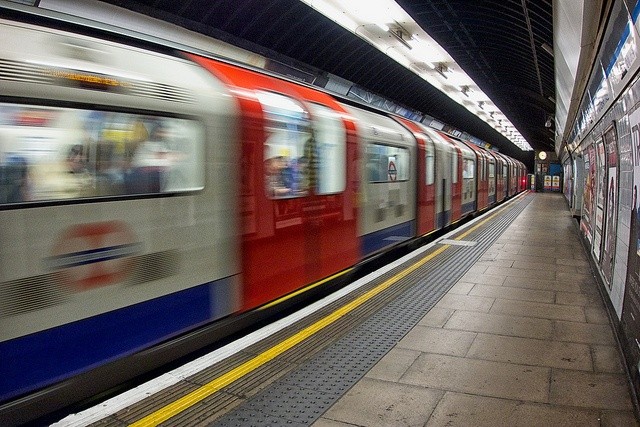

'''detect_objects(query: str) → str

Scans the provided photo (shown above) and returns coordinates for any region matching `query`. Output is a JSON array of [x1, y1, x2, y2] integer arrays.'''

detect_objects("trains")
[[1, 17, 528, 427]]
